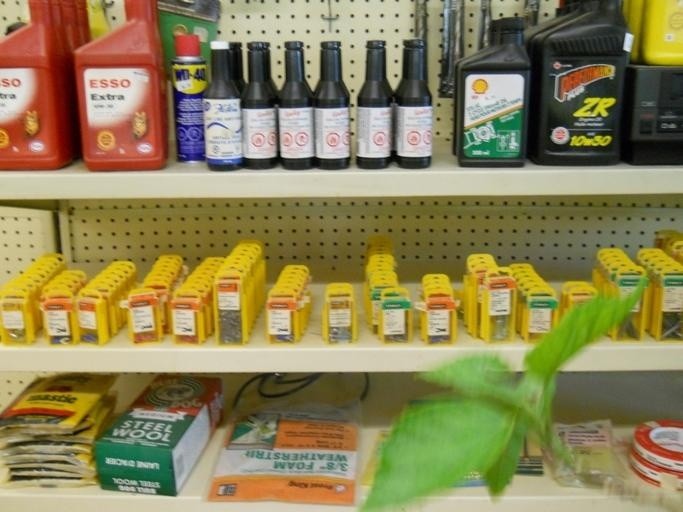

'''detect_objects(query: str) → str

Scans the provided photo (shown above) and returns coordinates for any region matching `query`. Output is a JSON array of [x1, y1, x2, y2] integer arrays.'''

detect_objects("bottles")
[[229, 41, 247, 94], [355, 40, 390, 169], [276, 41, 314, 169], [265, 41, 279, 97], [239, 42, 278, 169], [392, 41, 410, 162], [202, 41, 242, 171], [394, 39, 433, 168], [314, 40, 351, 170]]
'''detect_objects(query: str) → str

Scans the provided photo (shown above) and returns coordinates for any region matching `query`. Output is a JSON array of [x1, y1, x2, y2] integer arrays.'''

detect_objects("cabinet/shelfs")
[[0, 131, 683, 511]]
